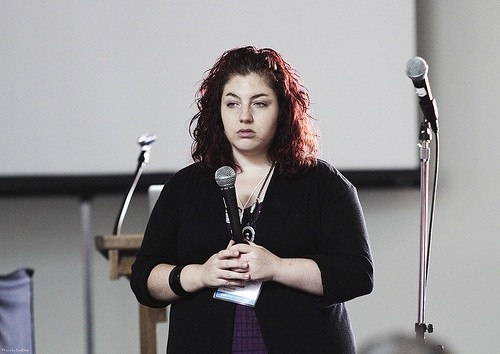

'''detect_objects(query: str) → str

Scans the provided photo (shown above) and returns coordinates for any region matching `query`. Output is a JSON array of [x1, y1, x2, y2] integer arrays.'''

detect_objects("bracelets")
[[169, 263, 193, 297]]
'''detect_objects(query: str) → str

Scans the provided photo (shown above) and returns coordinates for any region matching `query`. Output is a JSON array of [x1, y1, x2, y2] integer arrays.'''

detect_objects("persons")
[[130, 46, 374, 354]]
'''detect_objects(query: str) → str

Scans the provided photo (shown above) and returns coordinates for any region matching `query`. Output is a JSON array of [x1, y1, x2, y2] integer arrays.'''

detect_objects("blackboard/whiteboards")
[[1, 2, 422, 179]]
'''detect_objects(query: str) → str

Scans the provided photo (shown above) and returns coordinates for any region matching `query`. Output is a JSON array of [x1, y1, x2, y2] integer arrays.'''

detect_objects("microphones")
[[405, 57, 439, 134], [216, 167, 244, 246]]
